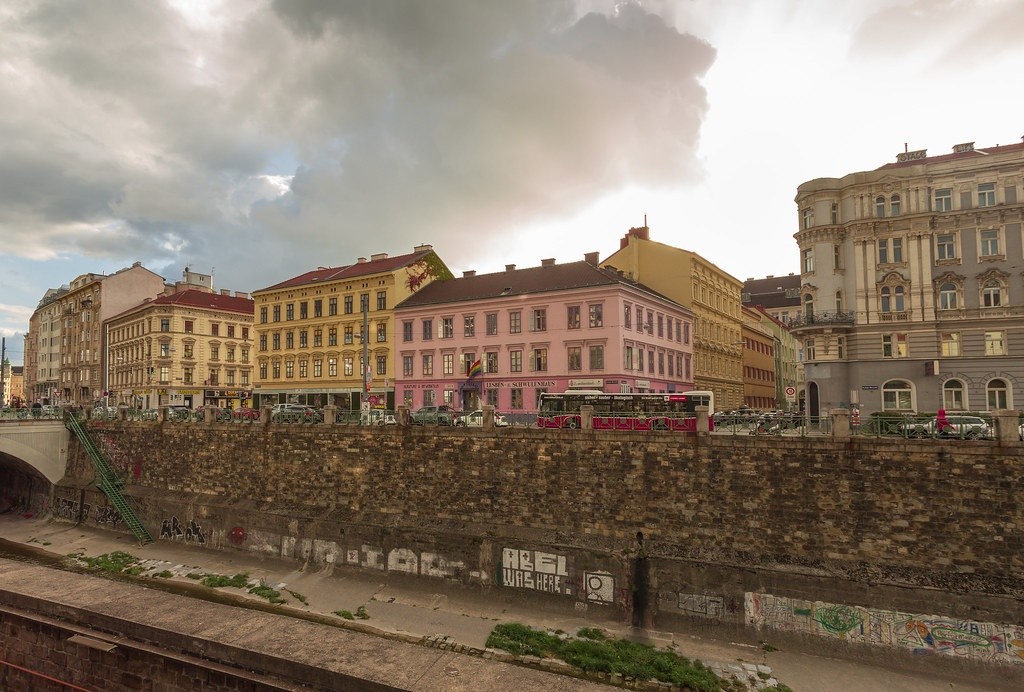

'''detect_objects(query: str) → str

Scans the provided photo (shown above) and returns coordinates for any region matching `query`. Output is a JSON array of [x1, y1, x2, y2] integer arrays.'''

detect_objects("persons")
[[759, 414, 777, 433], [935, 409, 956, 439]]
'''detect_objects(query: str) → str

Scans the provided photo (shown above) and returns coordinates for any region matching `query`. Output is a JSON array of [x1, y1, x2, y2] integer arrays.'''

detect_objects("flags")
[[467, 360, 481, 379]]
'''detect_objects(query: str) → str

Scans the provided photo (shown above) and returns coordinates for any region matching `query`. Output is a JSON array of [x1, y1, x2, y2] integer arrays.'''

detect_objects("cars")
[[713, 403, 805, 434], [1, 395, 510, 429]]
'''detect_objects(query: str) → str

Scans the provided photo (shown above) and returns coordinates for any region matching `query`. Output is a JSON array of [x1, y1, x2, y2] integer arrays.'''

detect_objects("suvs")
[[898, 414, 990, 440]]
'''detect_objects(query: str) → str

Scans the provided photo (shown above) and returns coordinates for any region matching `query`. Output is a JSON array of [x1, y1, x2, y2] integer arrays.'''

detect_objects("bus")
[[538, 388, 715, 436]]
